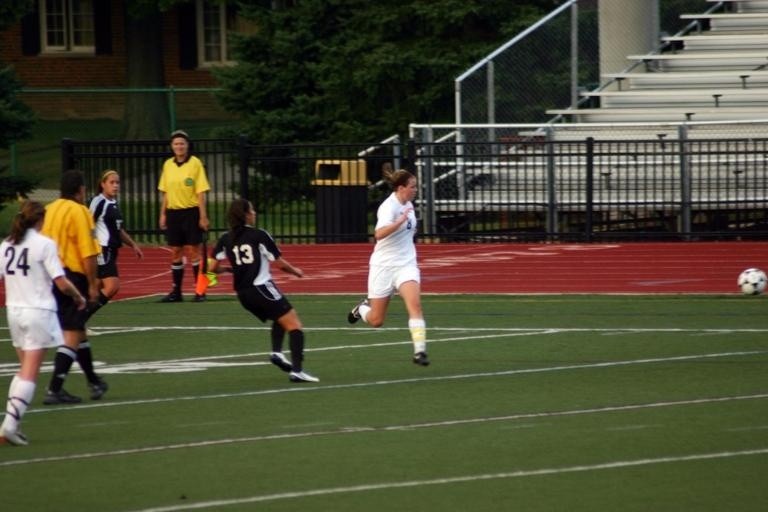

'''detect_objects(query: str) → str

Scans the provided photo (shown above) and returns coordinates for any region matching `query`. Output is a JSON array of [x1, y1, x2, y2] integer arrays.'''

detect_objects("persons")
[[0, 198, 87, 446], [345, 167, 430, 368], [155, 128, 211, 303], [39, 170, 109, 405], [207, 198, 321, 384], [84, 167, 145, 322]]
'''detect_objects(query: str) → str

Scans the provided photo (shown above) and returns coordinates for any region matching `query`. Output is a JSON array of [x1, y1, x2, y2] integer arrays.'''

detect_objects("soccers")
[[737, 267, 764, 294]]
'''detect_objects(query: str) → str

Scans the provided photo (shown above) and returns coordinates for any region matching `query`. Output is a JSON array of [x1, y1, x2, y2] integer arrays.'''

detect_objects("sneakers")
[[269, 351, 295, 373], [288, 370, 320, 383], [191, 294, 207, 303], [88, 380, 109, 400], [412, 352, 431, 367], [348, 299, 371, 324], [0, 427, 30, 447], [157, 292, 183, 303], [43, 387, 83, 406]]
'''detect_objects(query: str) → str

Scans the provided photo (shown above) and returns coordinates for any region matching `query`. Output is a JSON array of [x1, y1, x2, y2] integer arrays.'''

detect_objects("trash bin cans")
[[311, 159, 368, 244]]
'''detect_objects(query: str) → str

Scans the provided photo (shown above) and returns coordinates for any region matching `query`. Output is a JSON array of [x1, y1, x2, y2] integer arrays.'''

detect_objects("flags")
[[193, 255, 218, 295]]
